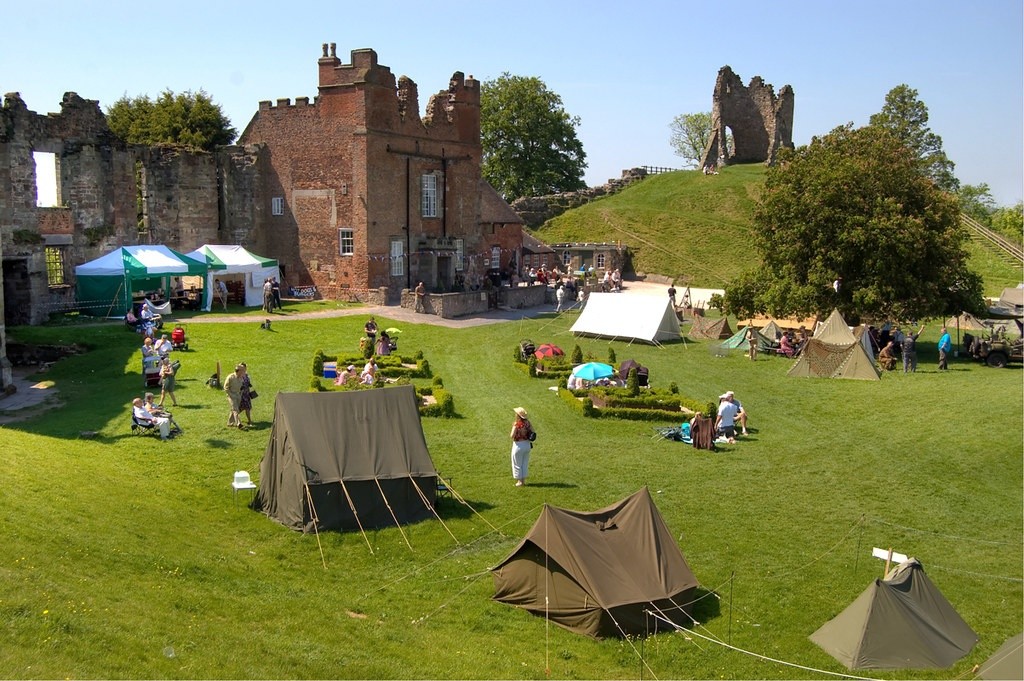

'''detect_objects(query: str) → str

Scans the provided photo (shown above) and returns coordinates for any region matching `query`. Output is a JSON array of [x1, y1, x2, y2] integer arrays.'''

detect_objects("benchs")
[[763, 338, 797, 357]]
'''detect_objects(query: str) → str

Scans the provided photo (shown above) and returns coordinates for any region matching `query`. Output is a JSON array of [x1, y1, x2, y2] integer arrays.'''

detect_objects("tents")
[[247, 384, 439, 535], [487, 479, 702, 642], [74, 244, 208, 320], [568, 292, 883, 391], [805, 555, 983, 672], [182, 244, 281, 313], [974, 632, 1024, 681]]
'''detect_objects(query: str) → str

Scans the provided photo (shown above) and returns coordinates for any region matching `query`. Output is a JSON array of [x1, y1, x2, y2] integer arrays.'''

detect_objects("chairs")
[[124, 315, 143, 331], [230, 469, 257, 511], [131, 411, 156, 437], [139, 308, 157, 328], [142, 356, 163, 388]]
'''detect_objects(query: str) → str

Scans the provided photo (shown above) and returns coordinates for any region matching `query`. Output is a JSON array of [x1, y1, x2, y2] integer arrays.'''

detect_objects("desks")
[[131, 280, 245, 310]]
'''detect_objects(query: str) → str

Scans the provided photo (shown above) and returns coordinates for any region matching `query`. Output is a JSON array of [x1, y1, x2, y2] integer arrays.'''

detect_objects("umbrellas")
[[534, 343, 566, 361], [385, 327, 403, 338]]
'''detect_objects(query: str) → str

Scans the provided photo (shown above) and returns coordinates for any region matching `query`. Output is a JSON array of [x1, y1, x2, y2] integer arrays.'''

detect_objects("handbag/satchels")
[[248, 384, 258, 399]]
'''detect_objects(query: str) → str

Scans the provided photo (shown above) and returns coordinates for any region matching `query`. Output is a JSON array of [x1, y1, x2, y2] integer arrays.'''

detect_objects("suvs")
[[969, 333, 1023, 368]]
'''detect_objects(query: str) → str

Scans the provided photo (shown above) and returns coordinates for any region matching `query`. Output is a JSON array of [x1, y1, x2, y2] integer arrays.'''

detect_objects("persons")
[[261, 276, 282, 314], [213, 279, 229, 310], [231, 361, 257, 428], [688, 390, 750, 445], [125, 276, 200, 444], [509, 406, 535, 487], [414, 282, 427, 313], [668, 284, 677, 307], [436, 256, 624, 314], [223, 364, 246, 430], [867, 315, 990, 376], [333, 316, 391, 387]]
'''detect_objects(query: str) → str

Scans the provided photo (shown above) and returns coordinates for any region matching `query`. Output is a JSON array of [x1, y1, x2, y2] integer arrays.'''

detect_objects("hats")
[[513, 407, 527, 420], [719, 394, 730, 400]]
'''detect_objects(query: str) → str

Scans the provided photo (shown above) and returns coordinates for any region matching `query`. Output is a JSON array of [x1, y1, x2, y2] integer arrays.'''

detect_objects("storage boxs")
[[681, 422, 692, 440], [323, 364, 336, 378]]
